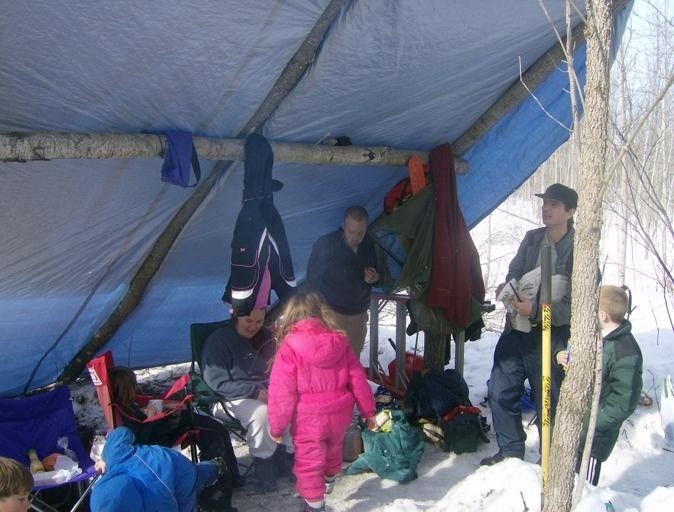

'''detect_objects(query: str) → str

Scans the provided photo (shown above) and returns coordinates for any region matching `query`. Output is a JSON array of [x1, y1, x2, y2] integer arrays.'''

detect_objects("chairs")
[[87, 350, 202, 469], [188, 319, 285, 481], [1, 380, 106, 512]]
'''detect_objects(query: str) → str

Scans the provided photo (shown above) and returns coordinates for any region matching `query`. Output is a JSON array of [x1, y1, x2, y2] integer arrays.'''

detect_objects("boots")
[[251, 444, 336, 512]]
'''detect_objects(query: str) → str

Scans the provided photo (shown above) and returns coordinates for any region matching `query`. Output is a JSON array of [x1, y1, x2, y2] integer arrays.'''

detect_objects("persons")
[[0, 454, 33, 512], [553, 283, 643, 486], [307, 205, 380, 365], [267, 287, 379, 510], [109, 365, 246, 489], [201, 305, 295, 493], [480, 182, 602, 466]]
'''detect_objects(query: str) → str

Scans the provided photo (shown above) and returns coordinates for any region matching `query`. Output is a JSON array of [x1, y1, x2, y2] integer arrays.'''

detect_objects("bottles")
[[25, 449, 45, 475]]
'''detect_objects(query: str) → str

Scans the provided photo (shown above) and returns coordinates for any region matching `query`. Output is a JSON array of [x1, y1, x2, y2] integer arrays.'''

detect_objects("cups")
[[146, 399, 162, 416]]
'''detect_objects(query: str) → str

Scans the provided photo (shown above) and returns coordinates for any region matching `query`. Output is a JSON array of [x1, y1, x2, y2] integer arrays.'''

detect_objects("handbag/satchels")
[[361, 410, 424, 481]]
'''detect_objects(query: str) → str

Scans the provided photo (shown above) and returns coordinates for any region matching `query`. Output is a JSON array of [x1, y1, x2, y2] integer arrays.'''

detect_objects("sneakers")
[[481, 453, 524, 466]]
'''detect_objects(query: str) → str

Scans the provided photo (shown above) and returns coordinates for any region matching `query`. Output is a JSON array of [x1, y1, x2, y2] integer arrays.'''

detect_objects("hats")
[[535, 184, 578, 207]]
